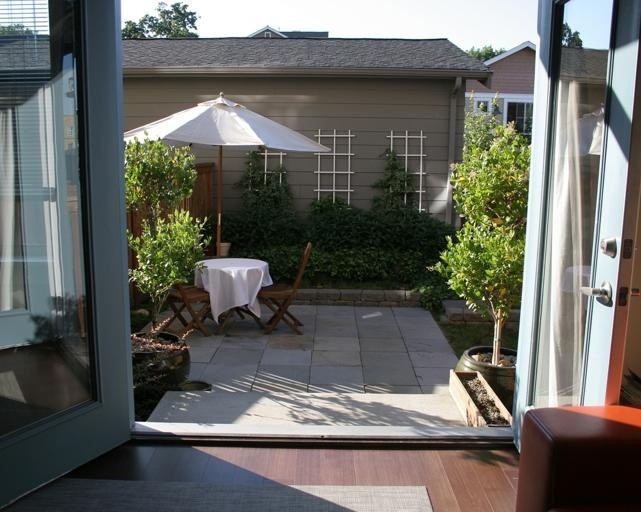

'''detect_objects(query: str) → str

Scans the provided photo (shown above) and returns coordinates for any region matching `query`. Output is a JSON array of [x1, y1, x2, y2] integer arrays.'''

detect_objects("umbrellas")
[[122, 90, 331, 259]]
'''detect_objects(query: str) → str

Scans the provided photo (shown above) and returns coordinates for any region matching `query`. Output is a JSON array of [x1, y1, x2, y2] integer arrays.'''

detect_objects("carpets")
[[0, 478, 434, 511]]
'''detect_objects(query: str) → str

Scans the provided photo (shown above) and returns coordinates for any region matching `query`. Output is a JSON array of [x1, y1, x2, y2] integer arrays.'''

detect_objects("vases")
[[424, 90, 531, 416], [216, 243, 232, 257]]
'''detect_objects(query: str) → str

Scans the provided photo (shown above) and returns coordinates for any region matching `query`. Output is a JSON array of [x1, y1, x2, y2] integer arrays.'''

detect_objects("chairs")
[[165, 281, 212, 338], [259, 242, 313, 335]]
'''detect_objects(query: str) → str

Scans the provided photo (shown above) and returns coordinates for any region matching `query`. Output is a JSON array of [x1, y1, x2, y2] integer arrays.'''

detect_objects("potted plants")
[[127, 206, 214, 402]]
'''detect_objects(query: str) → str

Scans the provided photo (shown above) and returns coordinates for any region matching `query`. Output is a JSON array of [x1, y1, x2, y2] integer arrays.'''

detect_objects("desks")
[[195, 258, 270, 335]]
